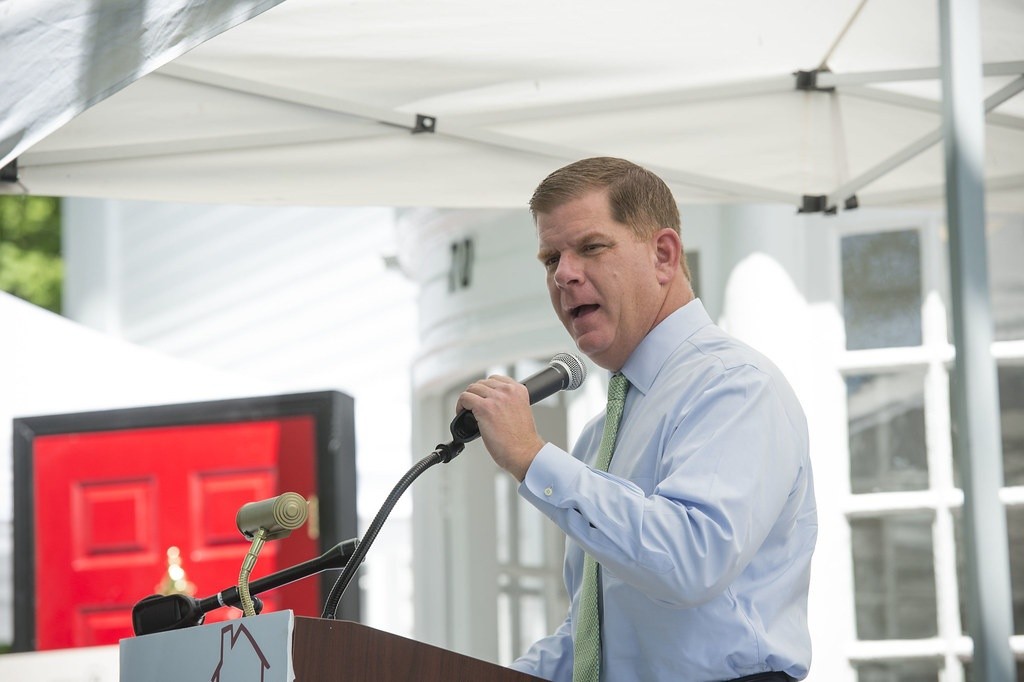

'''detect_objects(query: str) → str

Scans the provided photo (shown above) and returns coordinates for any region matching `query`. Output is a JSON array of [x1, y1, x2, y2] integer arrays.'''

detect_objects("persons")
[[508, 156, 817, 682]]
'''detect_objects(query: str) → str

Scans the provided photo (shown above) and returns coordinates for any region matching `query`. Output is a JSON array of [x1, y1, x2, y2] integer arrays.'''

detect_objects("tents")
[[0, 0, 1024, 682]]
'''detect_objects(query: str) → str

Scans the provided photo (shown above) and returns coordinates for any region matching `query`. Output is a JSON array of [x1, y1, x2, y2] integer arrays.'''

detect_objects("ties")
[[573, 374, 631, 682]]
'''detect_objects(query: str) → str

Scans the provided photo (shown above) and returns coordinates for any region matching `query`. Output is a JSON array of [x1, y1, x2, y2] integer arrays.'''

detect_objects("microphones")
[[133, 538, 367, 637], [451, 352, 586, 442]]
